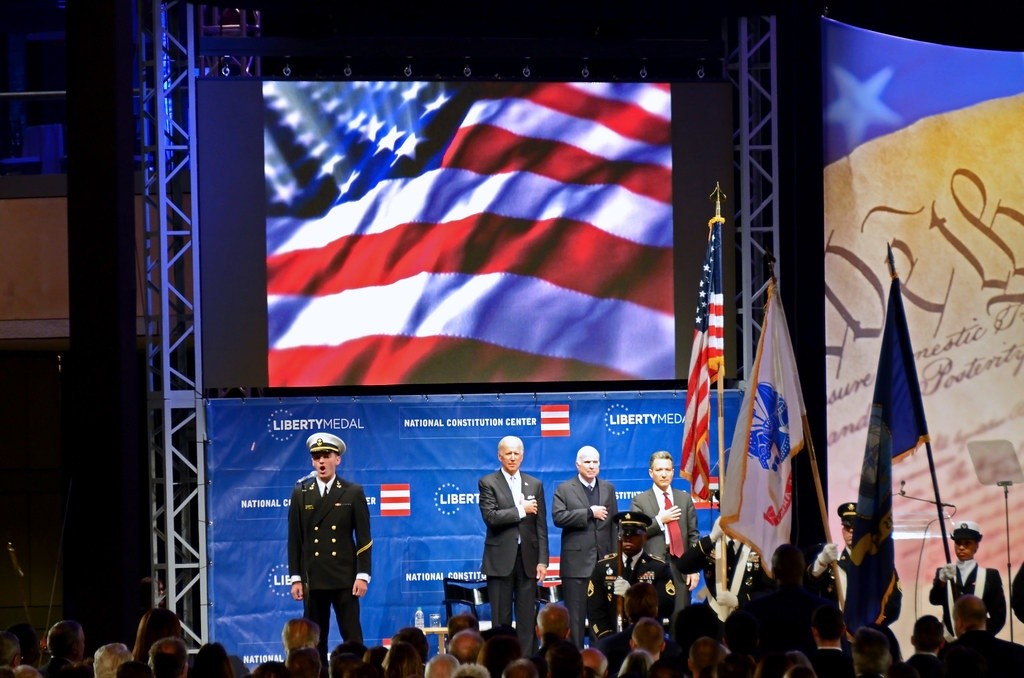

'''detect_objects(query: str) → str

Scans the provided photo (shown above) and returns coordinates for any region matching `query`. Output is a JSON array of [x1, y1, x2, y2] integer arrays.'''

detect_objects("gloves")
[[818, 544, 838, 564], [613, 576, 631, 597], [716, 590, 738, 609], [710, 516, 723, 543], [939, 564, 955, 581]]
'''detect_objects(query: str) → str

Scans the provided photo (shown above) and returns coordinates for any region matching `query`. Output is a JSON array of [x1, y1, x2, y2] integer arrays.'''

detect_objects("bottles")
[[414, 606, 424, 630]]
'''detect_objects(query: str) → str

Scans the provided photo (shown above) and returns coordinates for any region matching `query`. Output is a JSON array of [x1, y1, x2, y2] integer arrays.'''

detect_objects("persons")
[[1, 502, 1024, 678], [631, 450, 700, 644], [552, 446, 620, 652], [478, 435, 550, 659], [287, 432, 372, 678]]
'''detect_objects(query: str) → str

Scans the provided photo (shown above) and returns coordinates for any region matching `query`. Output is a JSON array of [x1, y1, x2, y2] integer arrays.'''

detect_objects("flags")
[[718, 284, 806, 579], [842, 279, 930, 642], [680, 216, 724, 503]]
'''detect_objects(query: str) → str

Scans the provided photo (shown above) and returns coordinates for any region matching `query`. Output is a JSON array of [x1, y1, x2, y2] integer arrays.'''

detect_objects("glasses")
[[19, 655, 23, 659]]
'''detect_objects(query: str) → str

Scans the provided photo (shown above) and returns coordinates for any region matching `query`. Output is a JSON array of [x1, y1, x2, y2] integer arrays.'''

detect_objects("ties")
[[626, 557, 633, 571], [510, 476, 515, 481], [728, 540, 735, 557], [663, 492, 684, 558], [588, 484, 592, 491], [322, 485, 328, 500]]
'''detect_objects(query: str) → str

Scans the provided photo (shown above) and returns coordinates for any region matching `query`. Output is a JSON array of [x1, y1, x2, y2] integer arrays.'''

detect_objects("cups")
[[429, 613, 441, 629]]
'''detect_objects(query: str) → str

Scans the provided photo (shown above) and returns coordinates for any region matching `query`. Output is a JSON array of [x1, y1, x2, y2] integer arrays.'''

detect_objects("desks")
[[422, 627, 448, 655]]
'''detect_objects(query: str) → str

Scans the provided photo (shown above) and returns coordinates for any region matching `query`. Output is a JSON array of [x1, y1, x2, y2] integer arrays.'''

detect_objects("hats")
[[612, 512, 653, 535], [951, 521, 983, 541], [306, 432, 346, 456], [838, 502, 857, 526]]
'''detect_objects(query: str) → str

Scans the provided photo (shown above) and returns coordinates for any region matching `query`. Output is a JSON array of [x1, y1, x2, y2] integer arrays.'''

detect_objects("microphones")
[[899, 480, 906, 495], [296, 471, 318, 485]]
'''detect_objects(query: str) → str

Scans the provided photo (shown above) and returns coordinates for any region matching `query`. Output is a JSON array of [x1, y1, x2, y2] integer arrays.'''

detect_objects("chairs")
[[442, 577, 566, 632]]
